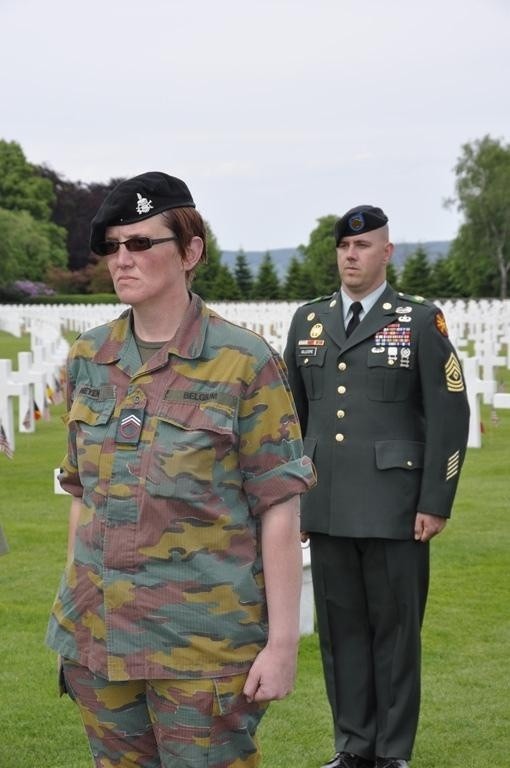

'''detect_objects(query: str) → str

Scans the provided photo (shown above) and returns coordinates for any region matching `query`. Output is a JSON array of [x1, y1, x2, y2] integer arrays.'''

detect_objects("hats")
[[335, 204, 388, 246], [88, 172, 196, 255]]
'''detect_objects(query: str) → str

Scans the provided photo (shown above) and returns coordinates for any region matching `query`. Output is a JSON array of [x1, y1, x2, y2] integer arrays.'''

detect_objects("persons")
[[281, 204, 469, 768], [47, 171, 316, 768]]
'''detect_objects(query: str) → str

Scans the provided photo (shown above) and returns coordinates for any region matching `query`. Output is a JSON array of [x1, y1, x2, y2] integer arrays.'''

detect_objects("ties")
[[346, 301, 362, 338]]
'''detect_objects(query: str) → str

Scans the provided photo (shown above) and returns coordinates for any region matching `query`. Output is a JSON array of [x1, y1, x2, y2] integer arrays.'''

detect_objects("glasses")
[[98, 236, 179, 255]]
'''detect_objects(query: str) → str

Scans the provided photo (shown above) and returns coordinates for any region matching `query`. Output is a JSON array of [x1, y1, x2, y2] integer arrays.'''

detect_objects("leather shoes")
[[322, 752, 410, 768]]
